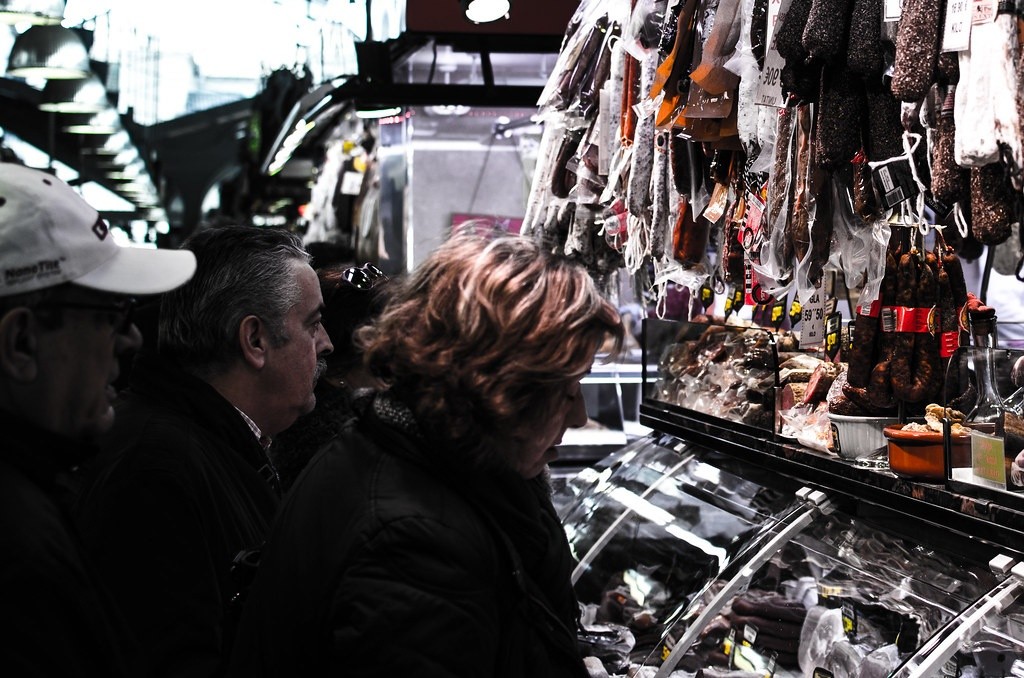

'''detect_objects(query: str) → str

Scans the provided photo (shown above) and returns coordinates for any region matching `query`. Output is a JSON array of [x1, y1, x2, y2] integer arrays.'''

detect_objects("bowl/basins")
[[827, 410, 927, 462], [884, 424, 974, 479]]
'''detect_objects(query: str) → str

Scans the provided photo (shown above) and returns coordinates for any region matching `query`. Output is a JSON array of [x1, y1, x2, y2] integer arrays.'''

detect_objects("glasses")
[[21, 298, 136, 335], [321, 261, 390, 314]]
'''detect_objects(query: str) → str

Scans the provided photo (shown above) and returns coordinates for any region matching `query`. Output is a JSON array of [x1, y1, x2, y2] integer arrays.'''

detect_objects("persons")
[[0, 164, 146, 678], [72, 222, 334, 678], [271, 261, 391, 484], [225, 218, 625, 678]]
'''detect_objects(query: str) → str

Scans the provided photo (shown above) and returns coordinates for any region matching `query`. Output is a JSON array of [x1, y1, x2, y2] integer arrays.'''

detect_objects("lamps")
[[7, 0, 170, 234]]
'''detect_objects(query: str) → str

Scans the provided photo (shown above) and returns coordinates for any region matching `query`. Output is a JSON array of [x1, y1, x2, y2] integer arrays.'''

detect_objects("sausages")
[[515, 0, 1024, 666]]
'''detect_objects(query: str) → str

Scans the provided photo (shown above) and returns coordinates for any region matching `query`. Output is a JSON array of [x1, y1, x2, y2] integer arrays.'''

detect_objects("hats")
[[0, 162, 197, 295]]
